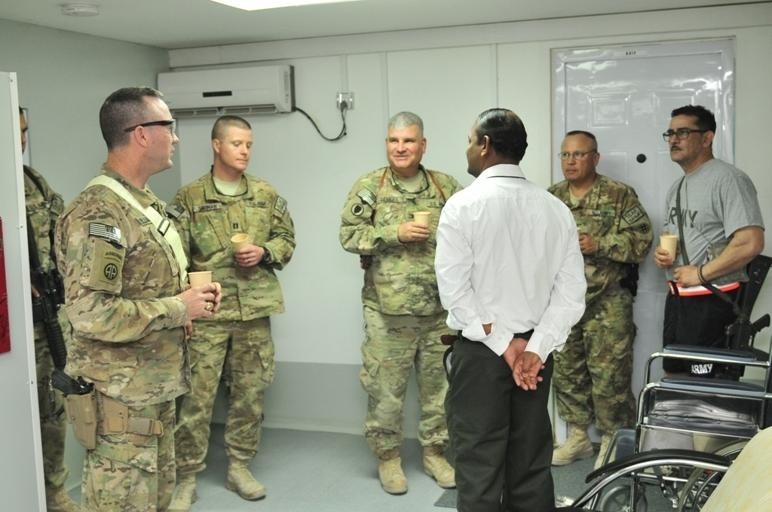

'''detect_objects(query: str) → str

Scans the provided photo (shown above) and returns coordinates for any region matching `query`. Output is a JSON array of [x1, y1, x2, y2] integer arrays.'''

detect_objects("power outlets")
[[336, 92, 354, 110]]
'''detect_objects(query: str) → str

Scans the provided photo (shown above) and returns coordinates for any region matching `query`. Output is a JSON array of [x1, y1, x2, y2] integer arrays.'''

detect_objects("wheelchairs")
[[555, 342, 772, 512]]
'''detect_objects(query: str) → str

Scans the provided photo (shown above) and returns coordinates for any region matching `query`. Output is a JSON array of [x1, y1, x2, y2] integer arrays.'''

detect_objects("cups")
[[230, 233, 250, 254], [659, 234, 677, 262], [187, 270, 213, 288], [412, 211, 431, 227]]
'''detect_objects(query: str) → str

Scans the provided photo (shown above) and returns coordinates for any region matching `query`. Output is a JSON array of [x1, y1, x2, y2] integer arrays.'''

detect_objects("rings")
[[206, 302, 212, 311]]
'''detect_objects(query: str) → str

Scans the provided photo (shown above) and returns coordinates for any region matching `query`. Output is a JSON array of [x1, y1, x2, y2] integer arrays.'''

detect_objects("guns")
[[52, 369, 93, 397], [619, 268, 637, 296], [26, 206, 66, 375], [723, 253, 771, 381]]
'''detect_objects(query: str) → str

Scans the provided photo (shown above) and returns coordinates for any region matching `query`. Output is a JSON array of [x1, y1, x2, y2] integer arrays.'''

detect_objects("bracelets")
[[698, 263, 706, 283]]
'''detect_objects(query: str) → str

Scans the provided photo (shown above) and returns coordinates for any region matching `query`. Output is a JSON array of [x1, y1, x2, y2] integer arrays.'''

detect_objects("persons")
[[339, 112, 465, 495], [19, 104, 82, 511], [160, 113, 295, 512], [542, 132, 653, 468], [435, 109, 587, 512], [655, 104, 765, 381], [54, 86, 222, 512]]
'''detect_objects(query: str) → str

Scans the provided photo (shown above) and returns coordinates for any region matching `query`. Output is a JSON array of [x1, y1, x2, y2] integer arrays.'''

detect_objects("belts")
[[441, 334, 459, 345]]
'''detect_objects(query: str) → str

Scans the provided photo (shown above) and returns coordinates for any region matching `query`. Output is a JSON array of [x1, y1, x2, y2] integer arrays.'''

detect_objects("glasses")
[[557, 150, 597, 160], [663, 129, 702, 142], [125, 119, 177, 135]]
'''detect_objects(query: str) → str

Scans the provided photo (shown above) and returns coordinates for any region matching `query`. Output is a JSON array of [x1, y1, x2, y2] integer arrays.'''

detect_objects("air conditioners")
[[156, 64, 295, 119]]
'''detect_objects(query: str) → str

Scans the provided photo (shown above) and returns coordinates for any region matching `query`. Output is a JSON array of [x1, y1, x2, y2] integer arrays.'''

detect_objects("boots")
[[377, 455, 407, 496], [227, 457, 265, 501], [550, 422, 595, 466], [168, 471, 199, 510], [594, 431, 616, 470], [421, 447, 457, 488]]
